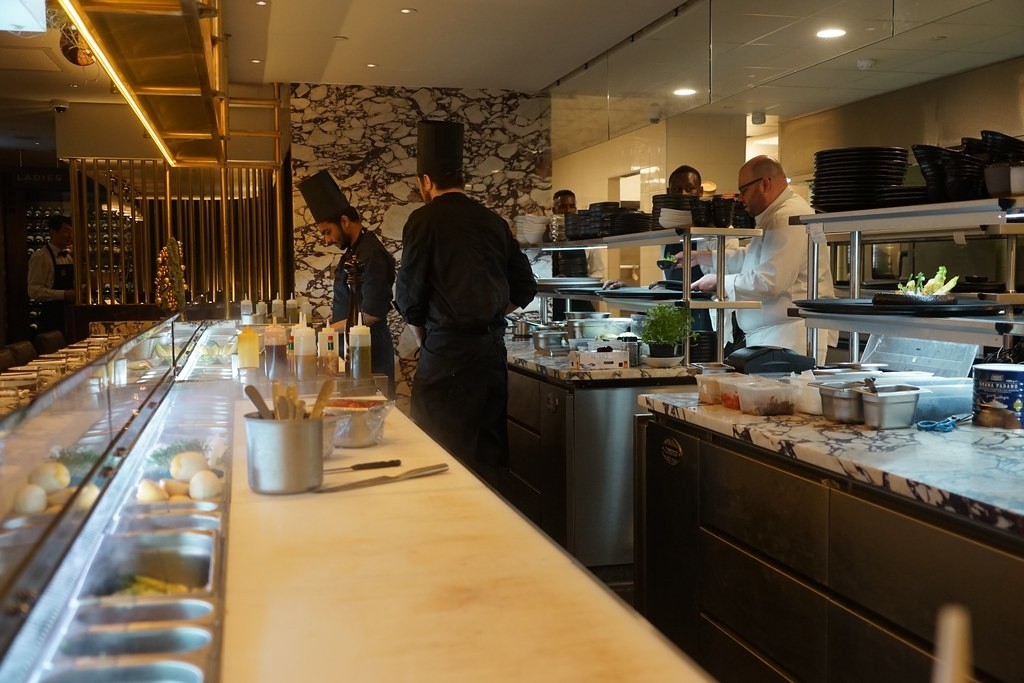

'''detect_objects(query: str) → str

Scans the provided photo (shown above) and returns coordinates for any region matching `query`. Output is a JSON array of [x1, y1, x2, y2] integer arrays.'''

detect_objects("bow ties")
[[58, 251, 68, 258]]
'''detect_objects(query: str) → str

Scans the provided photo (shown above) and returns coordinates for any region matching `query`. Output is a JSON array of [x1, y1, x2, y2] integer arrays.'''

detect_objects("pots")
[[504, 315, 542, 336], [527, 330, 569, 351]]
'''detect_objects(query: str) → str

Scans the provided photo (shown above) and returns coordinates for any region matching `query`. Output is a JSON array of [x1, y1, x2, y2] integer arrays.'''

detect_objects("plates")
[[613, 212, 653, 235], [809, 146, 929, 213], [651, 194, 698, 232], [689, 329, 717, 364]]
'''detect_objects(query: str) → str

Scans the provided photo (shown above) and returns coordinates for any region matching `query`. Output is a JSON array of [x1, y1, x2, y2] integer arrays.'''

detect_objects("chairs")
[[0, 331, 64, 375]]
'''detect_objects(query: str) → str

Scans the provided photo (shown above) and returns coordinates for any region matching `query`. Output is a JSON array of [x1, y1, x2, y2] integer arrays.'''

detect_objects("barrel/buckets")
[[971, 363, 1024, 429]]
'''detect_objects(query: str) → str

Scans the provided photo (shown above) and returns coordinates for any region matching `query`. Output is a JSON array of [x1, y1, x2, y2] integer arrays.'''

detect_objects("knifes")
[[323, 460, 401, 474], [311, 463, 450, 494]]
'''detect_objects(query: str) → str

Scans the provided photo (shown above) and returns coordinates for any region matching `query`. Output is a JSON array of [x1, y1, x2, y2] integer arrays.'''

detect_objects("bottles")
[[621, 337, 639, 367], [237, 311, 372, 379], [241, 292, 313, 329], [25, 206, 72, 333], [80, 209, 135, 305]]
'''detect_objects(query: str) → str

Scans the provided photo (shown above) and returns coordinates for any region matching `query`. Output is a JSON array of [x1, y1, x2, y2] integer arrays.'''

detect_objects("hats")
[[296, 169, 349, 224], [416, 120, 464, 177]]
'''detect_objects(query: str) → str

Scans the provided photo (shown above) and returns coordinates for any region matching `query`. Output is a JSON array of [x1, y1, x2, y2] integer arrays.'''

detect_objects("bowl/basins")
[[641, 355, 685, 368], [657, 260, 676, 271], [513, 213, 551, 245], [563, 311, 611, 323], [564, 201, 620, 241], [910, 130, 1024, 205], [320, 410, 337, 462], [689, 194, 756, 229], [308, 397, 395, 449]]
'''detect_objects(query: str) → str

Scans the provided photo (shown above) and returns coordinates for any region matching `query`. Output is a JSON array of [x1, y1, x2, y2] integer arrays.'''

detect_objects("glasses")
[[739, 178, 771, 195]]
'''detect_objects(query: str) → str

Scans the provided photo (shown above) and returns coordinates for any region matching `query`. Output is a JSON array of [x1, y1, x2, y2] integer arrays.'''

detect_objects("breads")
[[155, 342, 191, 355], [14, 461, 100, 514], [200, 340, 238, 355], [138, 450, 221, 501]]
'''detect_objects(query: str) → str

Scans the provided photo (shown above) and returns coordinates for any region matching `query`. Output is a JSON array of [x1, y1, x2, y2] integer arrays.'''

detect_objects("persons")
[[660, 165, 740, 331], [28, 216, 76, 311], [674, 155, 834, 366], [393, 120, 538, 490], [296, 170, 395, 402], [549, 190, 604, 311]]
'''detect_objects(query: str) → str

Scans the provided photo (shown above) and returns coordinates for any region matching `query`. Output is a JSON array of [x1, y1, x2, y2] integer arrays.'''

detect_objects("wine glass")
[[0, 317, 142, 422]]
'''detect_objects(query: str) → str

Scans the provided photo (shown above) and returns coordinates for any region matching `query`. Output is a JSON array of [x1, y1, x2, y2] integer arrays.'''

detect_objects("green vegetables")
[[661, 255, 676, 262], [44, 446, 103, 477], [151, 438, 210, 471]]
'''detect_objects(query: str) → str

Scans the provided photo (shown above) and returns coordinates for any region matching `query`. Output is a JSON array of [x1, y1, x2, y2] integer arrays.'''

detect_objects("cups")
[[243, 410, 324, 495]]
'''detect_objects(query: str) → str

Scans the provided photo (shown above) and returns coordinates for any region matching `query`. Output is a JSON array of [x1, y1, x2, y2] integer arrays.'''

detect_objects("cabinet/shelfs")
[[507, 195, 1024, 683]]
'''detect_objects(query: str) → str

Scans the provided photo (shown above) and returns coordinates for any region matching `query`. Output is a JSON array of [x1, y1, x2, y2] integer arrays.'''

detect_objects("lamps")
[[101, 172, 144, 222]]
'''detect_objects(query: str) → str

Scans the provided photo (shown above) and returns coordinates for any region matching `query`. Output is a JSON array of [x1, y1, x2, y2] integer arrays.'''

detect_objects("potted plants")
[[640, 302, 700, 357]]
